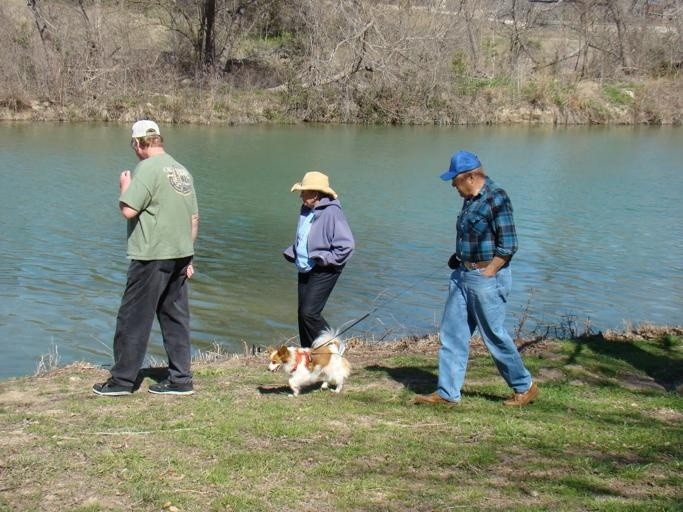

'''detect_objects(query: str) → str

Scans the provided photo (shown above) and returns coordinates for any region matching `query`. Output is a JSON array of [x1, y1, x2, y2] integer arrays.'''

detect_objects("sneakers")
[[415, 393, 448, 404], [93, 381, 134, 397], [149, 377, 193, 395], [503, 383, 538, 407]]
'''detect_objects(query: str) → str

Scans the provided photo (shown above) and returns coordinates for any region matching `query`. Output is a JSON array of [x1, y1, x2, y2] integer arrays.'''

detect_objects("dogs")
[[265, 325, 352, 399]]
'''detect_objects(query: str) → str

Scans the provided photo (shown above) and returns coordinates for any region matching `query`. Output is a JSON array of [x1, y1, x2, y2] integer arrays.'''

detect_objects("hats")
[[131, 120, 160, 137], [440, 150, 480, 180], [291, 172, 338, 200]]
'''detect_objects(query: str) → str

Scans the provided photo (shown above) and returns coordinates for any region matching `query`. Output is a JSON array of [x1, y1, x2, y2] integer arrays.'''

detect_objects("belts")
[[461, 262, 490, 270]]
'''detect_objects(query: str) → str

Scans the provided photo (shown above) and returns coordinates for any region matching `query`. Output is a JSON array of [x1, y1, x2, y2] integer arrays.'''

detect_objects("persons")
[[413, 149, 541, 407], [91, 119, 201, 396], [282, 170, 357, 347]]
